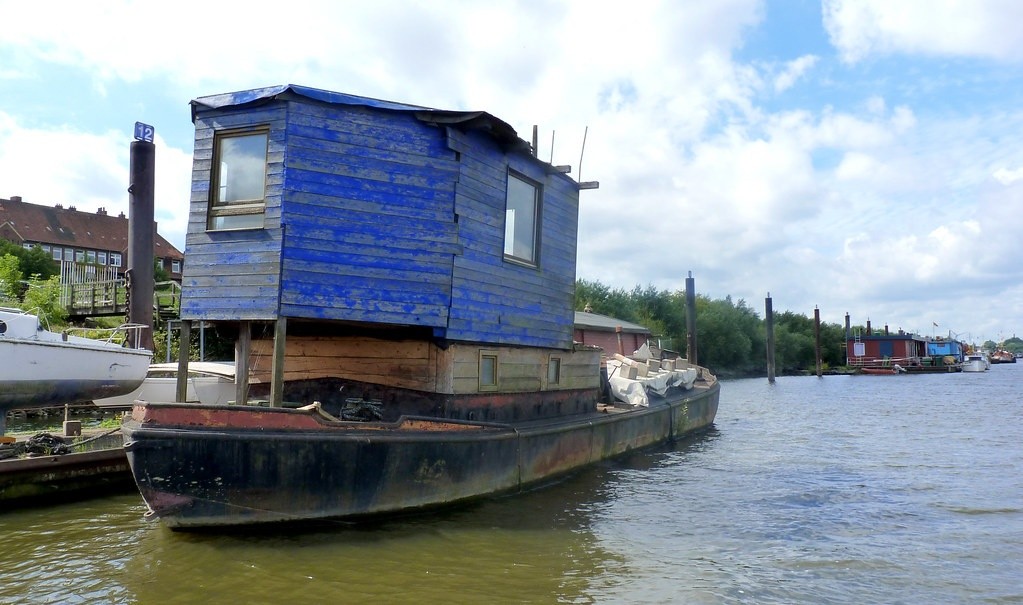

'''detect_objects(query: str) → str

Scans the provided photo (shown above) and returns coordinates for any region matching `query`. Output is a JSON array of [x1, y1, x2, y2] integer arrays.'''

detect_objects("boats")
[[0, 305, 154, 407], [118, 82, 720, 529], [91, 361, 237, 408], [962, 355, 986, 373], [989, 343, 1018, 364]]
